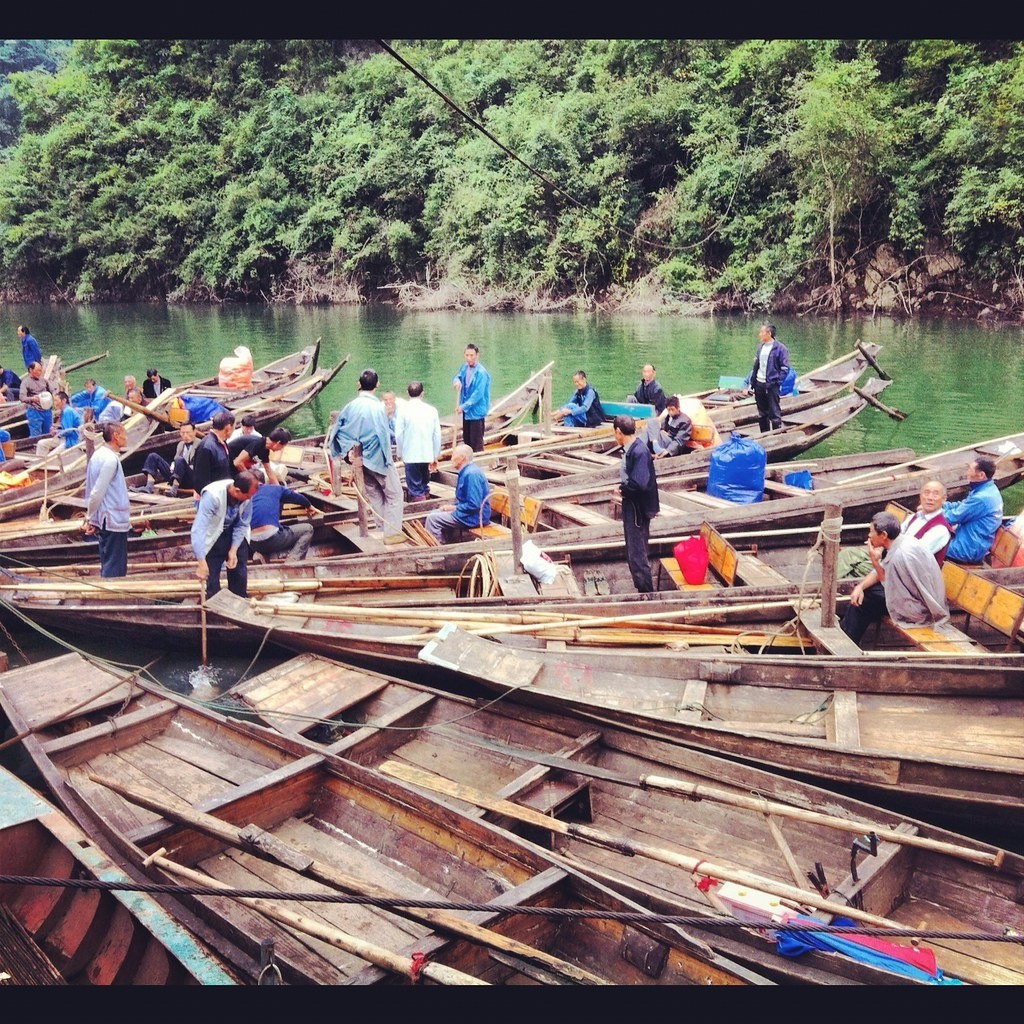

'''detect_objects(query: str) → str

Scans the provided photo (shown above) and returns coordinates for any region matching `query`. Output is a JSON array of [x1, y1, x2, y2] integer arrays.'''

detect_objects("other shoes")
[[165, 490, 176, 497], [139, 486, 151, 493], [284, 557, 296, 563], [384, 535, 408, 544], [253, 552, 266, 563]]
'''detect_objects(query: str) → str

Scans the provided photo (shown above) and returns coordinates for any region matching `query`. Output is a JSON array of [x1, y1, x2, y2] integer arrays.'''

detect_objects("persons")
[[242, 469, 314, 564], [0, 365, 22, 403], [191, 471, 258, 600], [329, 369, 409, 545], [645, 397, 693, 458], [875, 481, 956, 591], [750, 325, 789, 433], [17, 325, 42, 376], [840, 512, 944, 649], [916, 457, 1004, 564], [0, 429, 11, 463], [627, 363, 666, 416], [555, 371, 605, 427], [425, 444, 491, 544], [452, 344, 490, 456], [80, 423, 132, 578], [380, 390, 408, 445], [615, 415, 660, 593], [394, 382, 441, 502], [19, 361, 292, 497]]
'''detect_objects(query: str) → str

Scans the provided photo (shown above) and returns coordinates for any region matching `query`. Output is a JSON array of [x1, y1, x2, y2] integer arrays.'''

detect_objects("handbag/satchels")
[[519, 539, 556, 585], [674, 533, 708, 585], [785, 471, 812, 491], [779, 368, 798, 396]]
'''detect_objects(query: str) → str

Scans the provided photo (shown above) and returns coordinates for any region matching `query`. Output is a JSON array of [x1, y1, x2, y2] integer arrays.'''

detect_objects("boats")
[[0, 335, 1024, 986]]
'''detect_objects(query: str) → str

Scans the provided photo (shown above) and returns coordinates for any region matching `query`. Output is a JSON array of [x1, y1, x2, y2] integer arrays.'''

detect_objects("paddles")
[[232, 374, 324, 414], [23, 418, 130, 474], [836, 432, 1024, 484], [86, 771, 616, 985], [452, 388, 460, 451], [786, 402, 858, 432], [56, 440, 105, 475], [1, 502, 314, 544], [142, 845, 493, 986], [248, 596, 814, 651], [379, 759, 930, 947], [101, 388, 168, 425], [484, 404, 522, 422], [809, 462, 968, 496], [8, 557, 286, 574], [394, 426, 523, 468], [1, 576, 322, 602], [427, 720, 1007, 871]]
